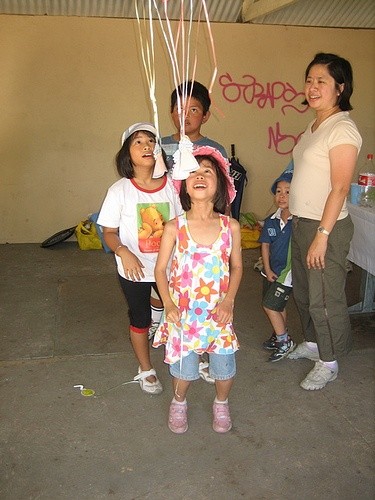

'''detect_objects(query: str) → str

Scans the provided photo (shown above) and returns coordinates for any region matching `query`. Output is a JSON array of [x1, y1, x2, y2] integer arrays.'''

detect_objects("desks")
[[346, 194, 375, 314]]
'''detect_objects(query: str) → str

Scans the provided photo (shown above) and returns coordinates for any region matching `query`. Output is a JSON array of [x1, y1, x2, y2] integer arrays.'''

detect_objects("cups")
[[351, 183, 361, 205]]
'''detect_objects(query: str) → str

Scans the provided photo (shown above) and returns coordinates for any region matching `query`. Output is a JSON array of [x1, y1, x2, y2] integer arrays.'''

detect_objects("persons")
[[258, 160, 298, 364], [95, 123, 215, 396], [152, 146, 244, 434], [285, 51, 363, 391], [147, 79, 225, 340]]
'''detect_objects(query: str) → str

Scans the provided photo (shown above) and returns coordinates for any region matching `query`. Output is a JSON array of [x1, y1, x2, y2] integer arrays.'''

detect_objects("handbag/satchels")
[[75, 220, 103, 250]]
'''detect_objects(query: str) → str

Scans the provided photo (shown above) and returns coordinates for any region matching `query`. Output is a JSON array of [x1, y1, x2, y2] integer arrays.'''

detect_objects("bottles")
[[357, 154, 375, 208]]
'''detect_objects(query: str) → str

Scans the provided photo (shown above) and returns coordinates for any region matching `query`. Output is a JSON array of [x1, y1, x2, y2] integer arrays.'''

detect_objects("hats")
[[121, 122, 157, 146], [271, 161, 294, 194], [173, 145, 237, 206]]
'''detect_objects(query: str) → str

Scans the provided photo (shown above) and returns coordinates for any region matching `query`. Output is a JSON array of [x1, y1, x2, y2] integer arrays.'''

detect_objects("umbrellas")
[[228, 143, 248, 223]]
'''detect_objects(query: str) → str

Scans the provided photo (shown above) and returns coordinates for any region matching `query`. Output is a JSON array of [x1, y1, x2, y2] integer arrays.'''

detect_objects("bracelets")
[[115, 244, 128, 257]]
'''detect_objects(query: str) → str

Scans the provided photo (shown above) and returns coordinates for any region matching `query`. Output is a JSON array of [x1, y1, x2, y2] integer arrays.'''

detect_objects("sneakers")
[[269, 339, 297, 363], [167, 401, 188, 433], [212, 401, 232, 433], [300, 360, 338, 391], [285, 341, 320, 361], [264, 334, 290, 348]]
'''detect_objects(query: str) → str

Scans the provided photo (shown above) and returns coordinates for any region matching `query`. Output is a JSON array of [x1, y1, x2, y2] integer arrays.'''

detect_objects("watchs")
[[317, 226, 330, 235]]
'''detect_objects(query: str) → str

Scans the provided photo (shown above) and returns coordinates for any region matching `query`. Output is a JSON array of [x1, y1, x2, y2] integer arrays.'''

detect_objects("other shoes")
[[196, 361, 216, 384], [145, 321, 159, 341], [134, 365, 163, 394], [254, 257, 264, 272]]
